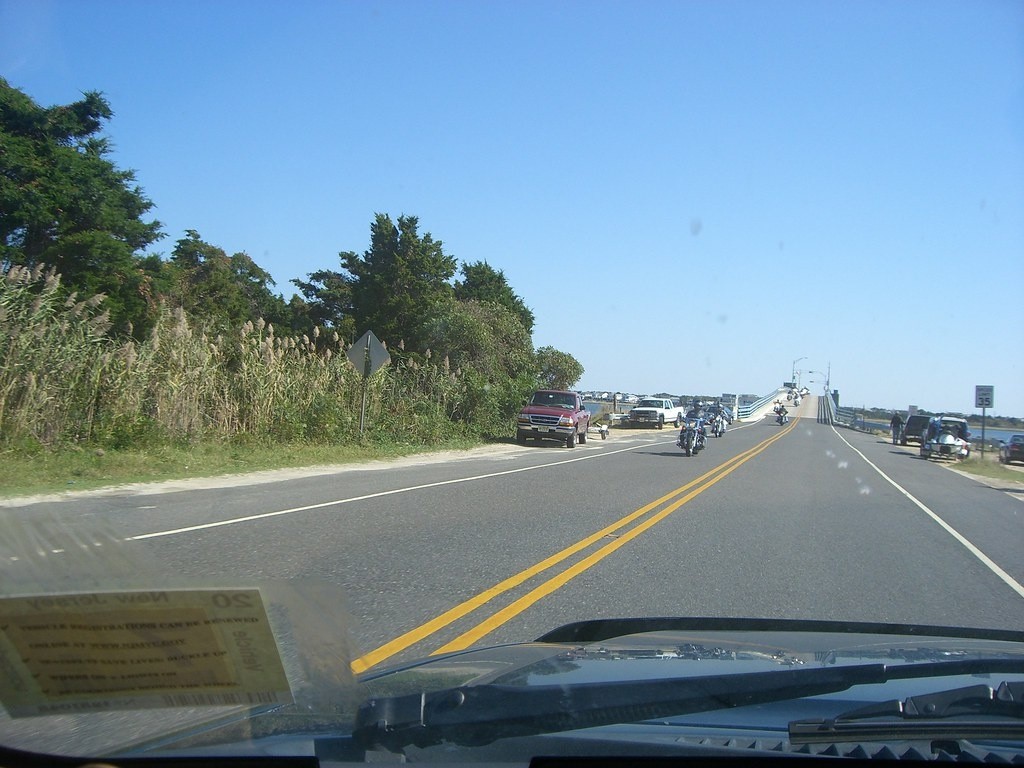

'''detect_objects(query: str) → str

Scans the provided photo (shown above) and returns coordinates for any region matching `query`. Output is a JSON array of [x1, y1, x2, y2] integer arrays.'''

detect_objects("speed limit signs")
[[975, 384, 995, 409]]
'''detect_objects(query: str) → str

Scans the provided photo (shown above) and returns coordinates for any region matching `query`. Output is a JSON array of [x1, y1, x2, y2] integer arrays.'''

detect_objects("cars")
[[687, 404, 733, 425], [998, 434, 1024, 465], [901, 413, 972, 460]]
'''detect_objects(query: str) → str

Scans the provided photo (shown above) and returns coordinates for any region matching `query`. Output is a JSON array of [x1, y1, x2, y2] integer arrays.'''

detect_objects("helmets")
[[718, 403, 724, 410], [693, 400, 703, 411]]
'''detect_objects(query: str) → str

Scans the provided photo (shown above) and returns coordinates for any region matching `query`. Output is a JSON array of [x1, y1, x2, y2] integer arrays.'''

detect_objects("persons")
[[776, 405, 788, 422], [890, 411, 905, 444], [676, 400, 710, 449], [772, 399, 782, 411], [710, 403, 728, 433]]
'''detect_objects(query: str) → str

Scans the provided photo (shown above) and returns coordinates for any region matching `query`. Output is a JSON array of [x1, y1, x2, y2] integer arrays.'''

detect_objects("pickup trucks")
[[516, 387, 591, 449], [627, 397, 685, 430]]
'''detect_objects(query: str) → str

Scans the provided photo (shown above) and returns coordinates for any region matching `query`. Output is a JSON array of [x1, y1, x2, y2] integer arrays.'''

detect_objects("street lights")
[[792, 356, 831, 389]]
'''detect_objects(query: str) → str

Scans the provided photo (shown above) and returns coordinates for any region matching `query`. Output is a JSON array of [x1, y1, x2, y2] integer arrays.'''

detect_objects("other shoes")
[[711, 429, 715, 433], [676, 442, 684, 446], [700, 444, 705, 449], [723, 429, 726, 433]]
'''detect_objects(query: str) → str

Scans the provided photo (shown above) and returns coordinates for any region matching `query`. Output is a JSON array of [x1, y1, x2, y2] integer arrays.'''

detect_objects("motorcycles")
[[773, 385, 810, 427], [676, 411, 705, 458], [708, 411, 729, 439]]
